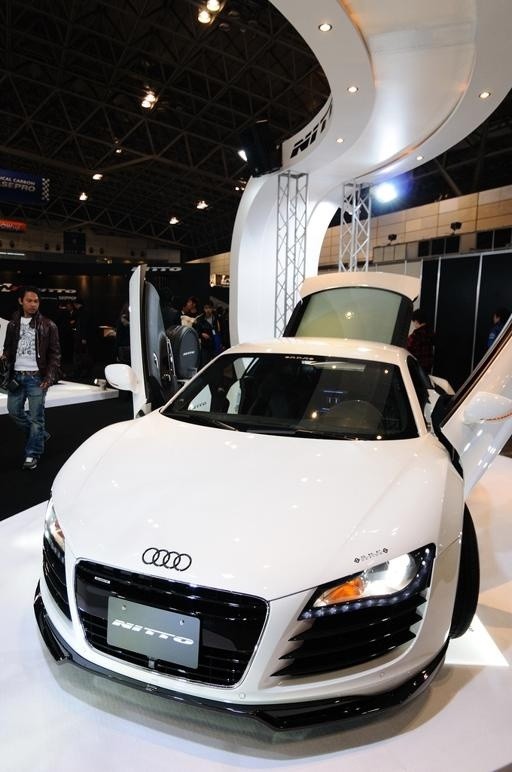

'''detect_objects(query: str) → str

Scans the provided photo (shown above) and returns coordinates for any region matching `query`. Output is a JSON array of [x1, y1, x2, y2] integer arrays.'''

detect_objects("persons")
[[165, 299, 229, 392], [117, 314, 129, 336], [407, 309, 436, 375], [487, 311, 503, 347], [54, 302, 94, 374], [0, 287, 62, 469]]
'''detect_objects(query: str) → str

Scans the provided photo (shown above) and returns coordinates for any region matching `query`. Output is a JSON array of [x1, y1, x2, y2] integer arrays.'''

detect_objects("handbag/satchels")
[[0, 374, 21, 396]]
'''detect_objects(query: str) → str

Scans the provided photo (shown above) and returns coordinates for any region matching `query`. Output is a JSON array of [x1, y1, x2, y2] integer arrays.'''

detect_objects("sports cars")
[[32, 263, 512, 732]]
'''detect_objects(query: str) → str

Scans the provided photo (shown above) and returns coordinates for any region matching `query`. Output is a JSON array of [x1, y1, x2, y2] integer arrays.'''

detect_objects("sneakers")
[[22, 457, 38, 472], [44, 431, 51, 442]]
